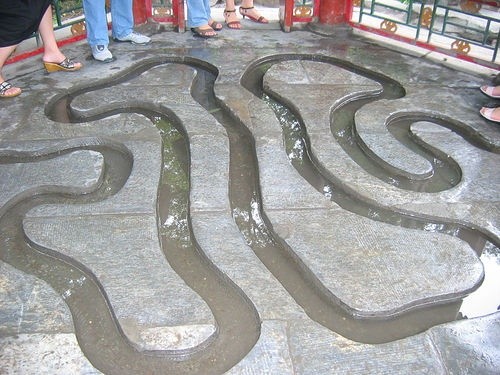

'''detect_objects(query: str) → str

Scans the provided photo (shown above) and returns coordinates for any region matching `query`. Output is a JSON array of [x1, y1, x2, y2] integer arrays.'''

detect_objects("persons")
[[186, 0, 223, 38], [223, 0, 269, 30], [479, 84, 500, 124], [82, 0, 152, 62], [0, 0, 83, 98]]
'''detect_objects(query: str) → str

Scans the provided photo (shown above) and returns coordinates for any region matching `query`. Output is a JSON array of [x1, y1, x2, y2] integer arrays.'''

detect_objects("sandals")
[[191, 26, 217, 37], [211, 21, 222, 30], [480, 85, 500, 98], [480, 107, 500, 123]]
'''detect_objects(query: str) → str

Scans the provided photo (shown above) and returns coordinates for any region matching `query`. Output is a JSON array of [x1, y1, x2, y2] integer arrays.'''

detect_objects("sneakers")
[[116, 31, 151, 45], [91, 44, 112, 61]]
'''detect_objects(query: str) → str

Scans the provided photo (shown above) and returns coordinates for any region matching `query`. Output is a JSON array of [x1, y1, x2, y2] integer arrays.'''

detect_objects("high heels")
[[0, 81, 20, 97], [240, 6, 268, 24], [223, 9, 241, 29], [44, 56, 80, 73]]
